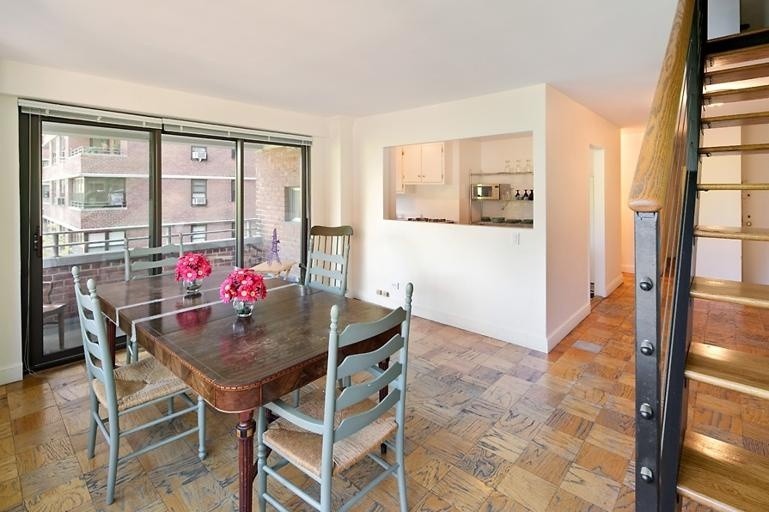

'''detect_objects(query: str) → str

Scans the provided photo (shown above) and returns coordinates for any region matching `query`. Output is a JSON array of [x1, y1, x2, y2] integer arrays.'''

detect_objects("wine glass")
[[503, 159, 533, 172], [510, 186, 533, 200]]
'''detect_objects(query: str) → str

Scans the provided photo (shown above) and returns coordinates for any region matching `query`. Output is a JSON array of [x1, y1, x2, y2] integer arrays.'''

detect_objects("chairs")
[[123, 234, 185, 366], [72, 266, 206, 506], [294, 225, 354, 407], [257, 282, 413, 512], [43, 280, 67, 353]]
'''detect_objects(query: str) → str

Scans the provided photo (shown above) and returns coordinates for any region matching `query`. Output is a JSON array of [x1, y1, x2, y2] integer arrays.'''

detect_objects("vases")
[[183, 276, 204, 294], [232, 300, 256, 317]]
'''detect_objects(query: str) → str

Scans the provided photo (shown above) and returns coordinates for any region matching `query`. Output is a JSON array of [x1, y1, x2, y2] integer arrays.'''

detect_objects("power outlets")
[[391, 282, 398, 290], [385, 291, 390, 297], [376, 288, 383, 295]]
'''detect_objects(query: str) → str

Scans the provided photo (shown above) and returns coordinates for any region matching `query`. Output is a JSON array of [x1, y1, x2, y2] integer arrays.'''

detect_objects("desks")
[[43, 303, 67, 349]]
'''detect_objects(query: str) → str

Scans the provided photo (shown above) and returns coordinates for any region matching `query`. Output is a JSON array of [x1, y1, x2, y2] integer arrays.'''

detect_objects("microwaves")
[[471, 183, 510, 201]]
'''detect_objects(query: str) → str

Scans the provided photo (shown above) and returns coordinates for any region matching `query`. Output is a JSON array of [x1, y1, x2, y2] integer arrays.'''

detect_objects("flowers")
[[175, 251, 212, 283], [219, 265, 268, 309]]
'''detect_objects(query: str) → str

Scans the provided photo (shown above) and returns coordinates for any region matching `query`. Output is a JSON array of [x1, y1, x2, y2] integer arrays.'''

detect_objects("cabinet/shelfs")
[[403, 143, 453, 188], [469, 168, 533, 228], [395, 146, 417, 196]]
